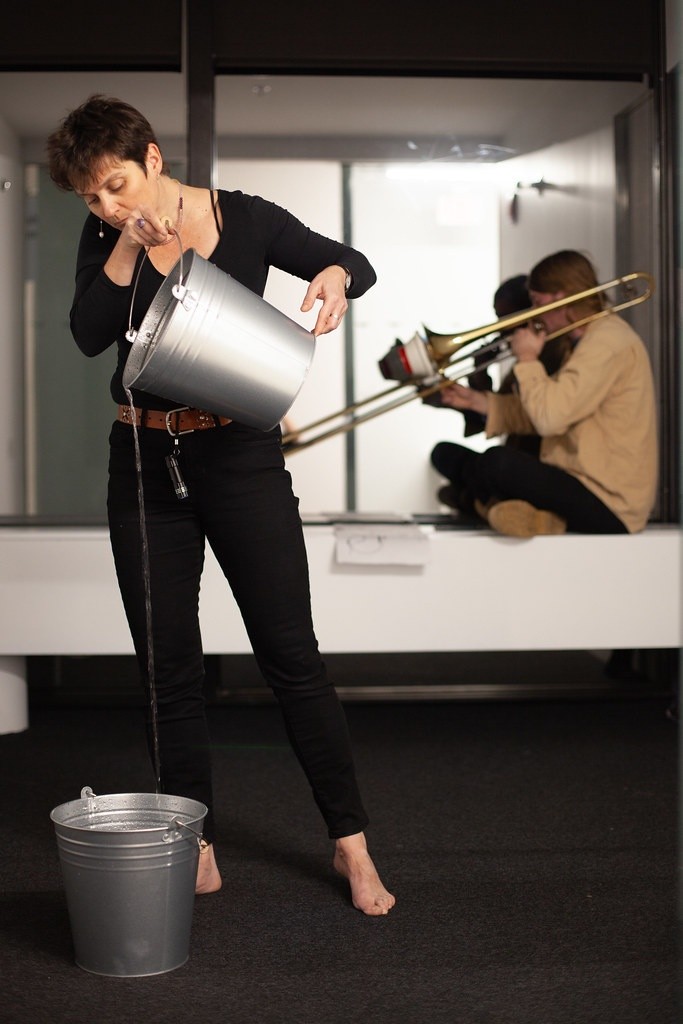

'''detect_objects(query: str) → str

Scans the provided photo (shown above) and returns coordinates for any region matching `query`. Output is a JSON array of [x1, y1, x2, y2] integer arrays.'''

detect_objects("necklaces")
[[161, 179, 184, 245]]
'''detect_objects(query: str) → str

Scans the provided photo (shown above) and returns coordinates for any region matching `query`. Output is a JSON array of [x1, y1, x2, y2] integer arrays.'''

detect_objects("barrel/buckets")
[[122, 216, 316, 432], [50, 786, 209, 978]]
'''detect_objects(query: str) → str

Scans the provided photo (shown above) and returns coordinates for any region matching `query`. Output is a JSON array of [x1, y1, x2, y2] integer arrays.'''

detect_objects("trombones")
[[275, 268, 654, 460]]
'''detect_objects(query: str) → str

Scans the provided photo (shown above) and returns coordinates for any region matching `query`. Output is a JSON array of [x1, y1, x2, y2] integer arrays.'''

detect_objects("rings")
[[329, 314, 340, 320], [136, 218, 146, 228]]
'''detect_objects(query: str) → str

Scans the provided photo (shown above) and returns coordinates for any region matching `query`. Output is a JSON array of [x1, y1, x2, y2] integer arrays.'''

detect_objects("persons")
[[46, 93, 397, 916], [415, 249, 659, 539], [417, 276, 560, 522]]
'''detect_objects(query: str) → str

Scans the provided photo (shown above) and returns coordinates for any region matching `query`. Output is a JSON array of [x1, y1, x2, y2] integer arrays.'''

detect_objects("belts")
[[116, 405, 232, 431]]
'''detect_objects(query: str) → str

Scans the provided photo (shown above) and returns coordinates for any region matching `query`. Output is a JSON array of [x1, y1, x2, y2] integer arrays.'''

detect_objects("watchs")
[[336, 263, 352, 291]]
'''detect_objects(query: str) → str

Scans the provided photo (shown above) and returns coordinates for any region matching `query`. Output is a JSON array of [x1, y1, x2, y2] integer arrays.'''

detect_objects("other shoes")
[[474, 497, 567, 539]]
